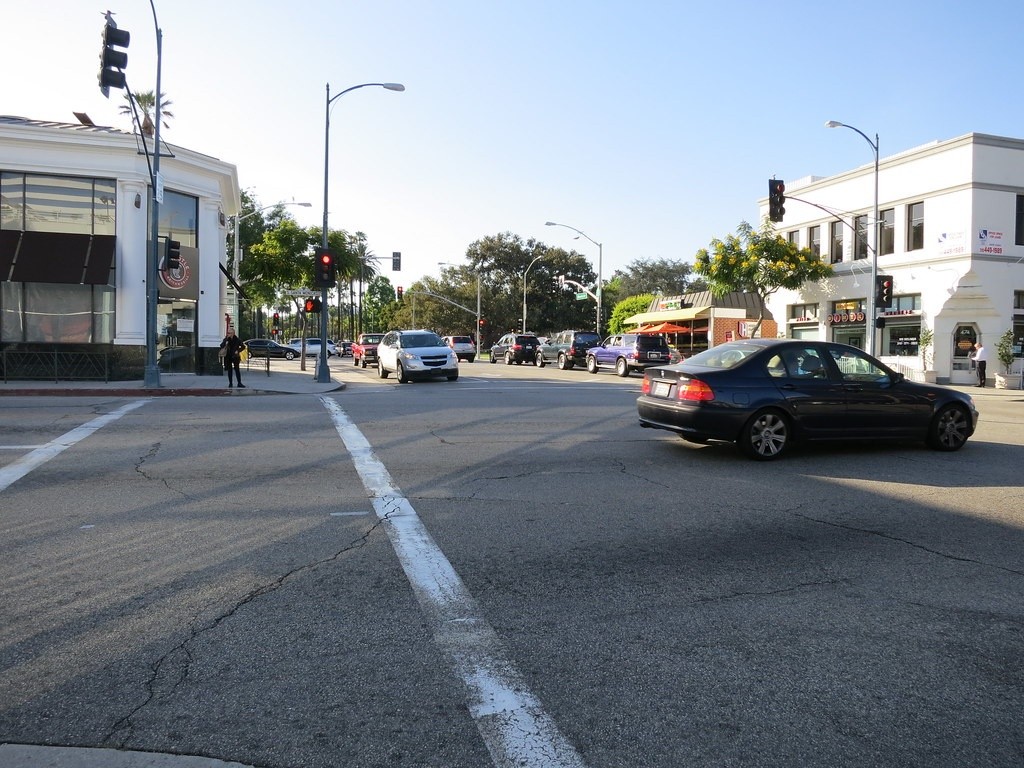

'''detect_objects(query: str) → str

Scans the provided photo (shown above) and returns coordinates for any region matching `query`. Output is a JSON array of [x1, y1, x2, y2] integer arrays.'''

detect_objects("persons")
[[220, 328, 246, 387], [971, 343, 987, 387]]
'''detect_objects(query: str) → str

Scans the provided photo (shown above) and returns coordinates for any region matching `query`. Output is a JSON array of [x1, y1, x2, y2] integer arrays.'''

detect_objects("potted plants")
[[993, 328, 1022, 390], [913, 323, 938, 383]]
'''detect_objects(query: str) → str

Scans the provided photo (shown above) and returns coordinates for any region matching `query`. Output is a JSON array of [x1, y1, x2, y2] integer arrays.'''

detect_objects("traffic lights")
[[100, 12, 130, 100], [273, 312, 279, 327], [768, 178, 786, 222], [304, 299, 315, 312], [272, 330, 276, 334], [397, 287, 402, 299], [479, 320, 484, 330], [164, 238, 180, 271], [591, 309, 597, 326], [315, 249, 335, 288], [517, 319, 522, 330], [876, 275, 893, 308], [878, 317, 885, 328]]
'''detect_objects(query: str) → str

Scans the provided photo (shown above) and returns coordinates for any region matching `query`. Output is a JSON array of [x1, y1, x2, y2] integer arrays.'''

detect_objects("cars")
[[440, 336, 476, 364], [287, 338, 356, 358], [488, 332, 542, 367], [669, 347, 685, 365], [635, 338, 979, 462], [241, 339, 302, 360]]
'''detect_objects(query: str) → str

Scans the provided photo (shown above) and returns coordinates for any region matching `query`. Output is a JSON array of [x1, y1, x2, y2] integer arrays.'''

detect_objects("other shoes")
[[229, 383, 233, 387], [237, 383, 246, 388], [974, 385, 982, 387]]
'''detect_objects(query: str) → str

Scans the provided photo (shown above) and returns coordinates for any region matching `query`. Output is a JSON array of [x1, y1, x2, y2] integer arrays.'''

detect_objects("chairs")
[[707, 359, 722, 366], [797, 355, 821, 377], [722, 360, 738, 366]]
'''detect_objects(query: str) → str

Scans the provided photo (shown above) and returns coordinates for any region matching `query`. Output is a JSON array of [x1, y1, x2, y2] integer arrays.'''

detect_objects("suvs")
[[585, 333, 671, 378], [535, 330, 603, 370], [375, 327, 460, 383], [284, 338, 337, 359]]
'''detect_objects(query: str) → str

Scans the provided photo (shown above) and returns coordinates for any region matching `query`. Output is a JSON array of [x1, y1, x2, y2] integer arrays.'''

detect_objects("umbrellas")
[[628, 324, 690, 346]]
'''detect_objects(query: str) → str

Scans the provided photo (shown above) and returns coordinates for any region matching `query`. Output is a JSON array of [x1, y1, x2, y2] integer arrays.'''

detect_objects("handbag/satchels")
[[217, 346, 227, 357]]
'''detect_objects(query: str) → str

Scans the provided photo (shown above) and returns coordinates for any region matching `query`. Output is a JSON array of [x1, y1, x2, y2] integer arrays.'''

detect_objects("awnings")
[[623, 306, 711, 324]]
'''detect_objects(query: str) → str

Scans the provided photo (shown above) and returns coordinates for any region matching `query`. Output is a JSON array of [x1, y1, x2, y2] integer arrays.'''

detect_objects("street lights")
[[523, 255, 547, 334], [544, 221, 603, 334], [232, 202, 313, 336], [316, 82, 406, 378], [825, 119, 879, 373], [437, 262, 481, 360]]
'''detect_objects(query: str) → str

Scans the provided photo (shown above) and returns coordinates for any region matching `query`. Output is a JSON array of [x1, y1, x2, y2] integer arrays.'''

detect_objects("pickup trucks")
[[349, 333, 388, 368]]
